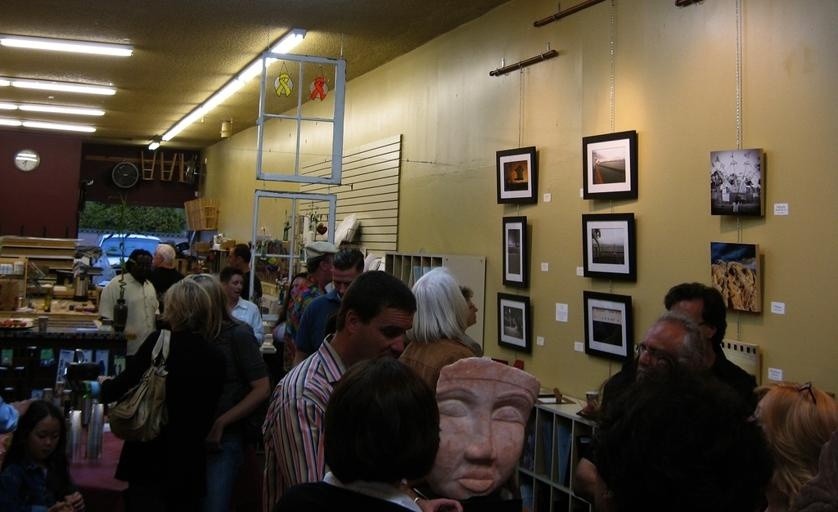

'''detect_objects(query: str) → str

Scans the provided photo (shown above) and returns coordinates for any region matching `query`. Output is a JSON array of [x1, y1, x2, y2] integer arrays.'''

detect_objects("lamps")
[[0, 35, 134, 57], [162, 29, 307, 140], [148, 142, 160, 151], [0, 118, 96, 137], [0, 77, 117, 96], [0, 102, 106, 117]]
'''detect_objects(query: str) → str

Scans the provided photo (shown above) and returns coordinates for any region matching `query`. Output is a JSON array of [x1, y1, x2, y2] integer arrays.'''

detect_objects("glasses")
[[797, 382, 817, 406]]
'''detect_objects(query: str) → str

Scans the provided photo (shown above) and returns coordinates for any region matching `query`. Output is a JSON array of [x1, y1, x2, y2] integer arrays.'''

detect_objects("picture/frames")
[[583, 290, 632, 362], [582, 213, 635, 282], [496, 146, 537, 204], [582, 130, 637, 200], [497, 293, 532, 356], [502, 216, 528, 289]]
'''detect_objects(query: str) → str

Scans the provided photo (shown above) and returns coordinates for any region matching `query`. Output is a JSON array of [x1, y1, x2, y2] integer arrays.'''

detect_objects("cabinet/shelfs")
[[385, 252, 486, 355], [516, 395, 595, 512]]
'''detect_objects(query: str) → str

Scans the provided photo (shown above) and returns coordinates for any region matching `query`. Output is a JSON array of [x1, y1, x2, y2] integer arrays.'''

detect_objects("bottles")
[[44, 288, 51, 312], [42, 381, 92, 427], [278, 290, 284, 304]]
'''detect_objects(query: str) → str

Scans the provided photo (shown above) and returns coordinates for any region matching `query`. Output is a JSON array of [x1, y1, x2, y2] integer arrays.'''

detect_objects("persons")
[[2, 242, 364, 512], [261, 270, 465, 511], [574, 282, 837, 512], [266, 358, 465, 512], [425, 357, 541, 501], [459, 286, 482, 357], [397, 265, 523, 511]]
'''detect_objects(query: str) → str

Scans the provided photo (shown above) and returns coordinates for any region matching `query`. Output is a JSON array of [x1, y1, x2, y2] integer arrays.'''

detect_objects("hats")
[[304, 241, 340, 258]]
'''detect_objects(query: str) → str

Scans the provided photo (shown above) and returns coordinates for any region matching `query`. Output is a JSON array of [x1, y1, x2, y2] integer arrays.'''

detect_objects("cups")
[[38, 316, 48, 333], [585, 391, 601, 407]]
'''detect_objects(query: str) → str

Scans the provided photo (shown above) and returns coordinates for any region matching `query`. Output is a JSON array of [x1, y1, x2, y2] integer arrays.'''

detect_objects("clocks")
[[16, 151, 40, 171]]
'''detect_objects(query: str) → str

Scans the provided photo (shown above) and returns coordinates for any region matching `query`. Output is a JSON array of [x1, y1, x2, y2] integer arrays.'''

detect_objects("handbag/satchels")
[[109, 358, 168, 442]]
